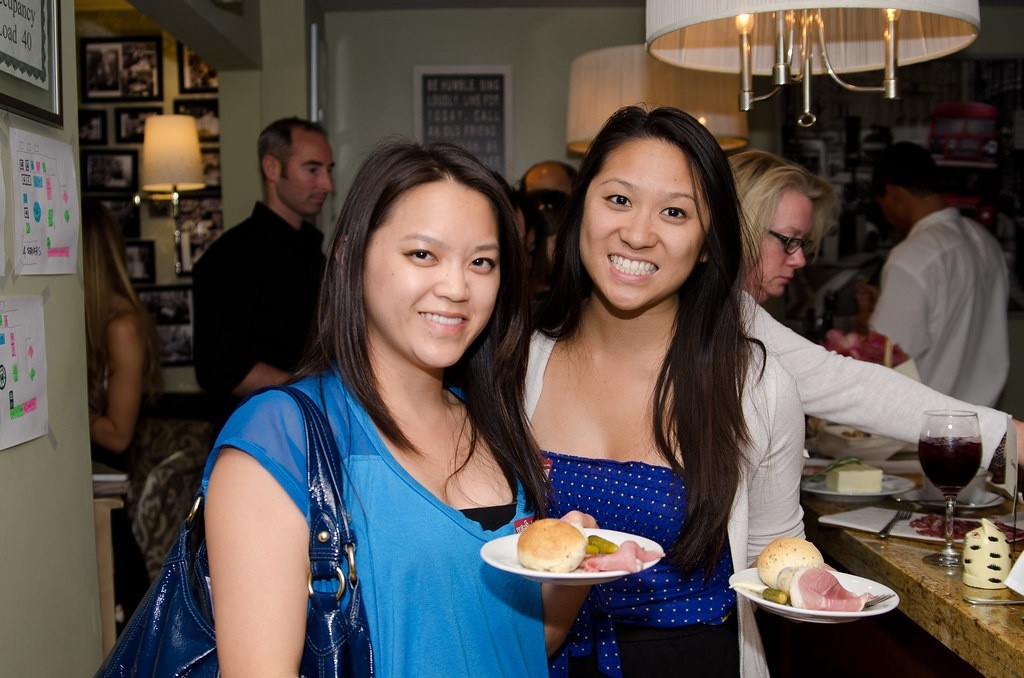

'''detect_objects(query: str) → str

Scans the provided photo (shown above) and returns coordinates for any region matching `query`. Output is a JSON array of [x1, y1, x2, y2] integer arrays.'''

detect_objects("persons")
[[520, 107, 826, 678], [77, 115, 1023, 617], [102, 147, 597, 678]]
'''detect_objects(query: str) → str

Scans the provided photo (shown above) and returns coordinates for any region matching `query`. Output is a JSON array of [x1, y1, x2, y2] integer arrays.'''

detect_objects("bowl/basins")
[[820, 423, 900, 449]]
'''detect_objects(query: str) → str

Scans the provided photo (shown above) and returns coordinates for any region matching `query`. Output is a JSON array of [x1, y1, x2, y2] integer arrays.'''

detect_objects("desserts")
[[825, 462, 885, 493]]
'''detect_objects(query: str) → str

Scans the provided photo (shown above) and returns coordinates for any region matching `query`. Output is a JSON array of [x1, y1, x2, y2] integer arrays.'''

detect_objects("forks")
[[877, 509, 913, 538]]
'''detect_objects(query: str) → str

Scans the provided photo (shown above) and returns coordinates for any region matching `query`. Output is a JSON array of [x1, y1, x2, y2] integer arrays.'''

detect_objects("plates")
[[728, 565, 901, 624], [799, 473, 917, 502], [817, 506, 1023, 544], [480, 527, 664, 586]]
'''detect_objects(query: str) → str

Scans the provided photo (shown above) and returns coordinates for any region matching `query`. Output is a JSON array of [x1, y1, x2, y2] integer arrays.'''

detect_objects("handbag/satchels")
[[94, 385, 376, 678]]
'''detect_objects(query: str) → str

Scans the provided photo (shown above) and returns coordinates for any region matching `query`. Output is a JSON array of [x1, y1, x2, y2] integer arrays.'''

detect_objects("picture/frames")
[[0, 0, 66, 130], [78, 31, 221, 368]]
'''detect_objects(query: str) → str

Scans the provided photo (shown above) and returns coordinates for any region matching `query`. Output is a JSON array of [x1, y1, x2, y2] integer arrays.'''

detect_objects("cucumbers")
[[584, 534, 618, 555], [762, 588, 788, 605]]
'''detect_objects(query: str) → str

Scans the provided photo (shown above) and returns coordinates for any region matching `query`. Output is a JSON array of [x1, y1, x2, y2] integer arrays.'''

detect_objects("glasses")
[[769, 230, 814, 258]]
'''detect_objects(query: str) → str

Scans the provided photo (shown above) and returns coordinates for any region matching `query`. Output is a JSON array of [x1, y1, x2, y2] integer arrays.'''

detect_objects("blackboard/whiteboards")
[[411, 64, 513, 187]]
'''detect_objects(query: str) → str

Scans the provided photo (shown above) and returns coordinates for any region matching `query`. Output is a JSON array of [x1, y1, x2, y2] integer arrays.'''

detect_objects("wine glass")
[[918, 410, 982, 571]]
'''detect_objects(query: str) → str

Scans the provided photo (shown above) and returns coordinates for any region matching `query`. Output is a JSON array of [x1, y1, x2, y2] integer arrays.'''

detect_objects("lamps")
[[567, 46, 751, 155], [138, 114, 206, 277], [646, 0, 983, 129]]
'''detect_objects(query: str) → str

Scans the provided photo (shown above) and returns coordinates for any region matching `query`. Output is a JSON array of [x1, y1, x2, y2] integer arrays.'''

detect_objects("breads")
[[757, 537, 824, 589], [516, 517, 587, 574]]
